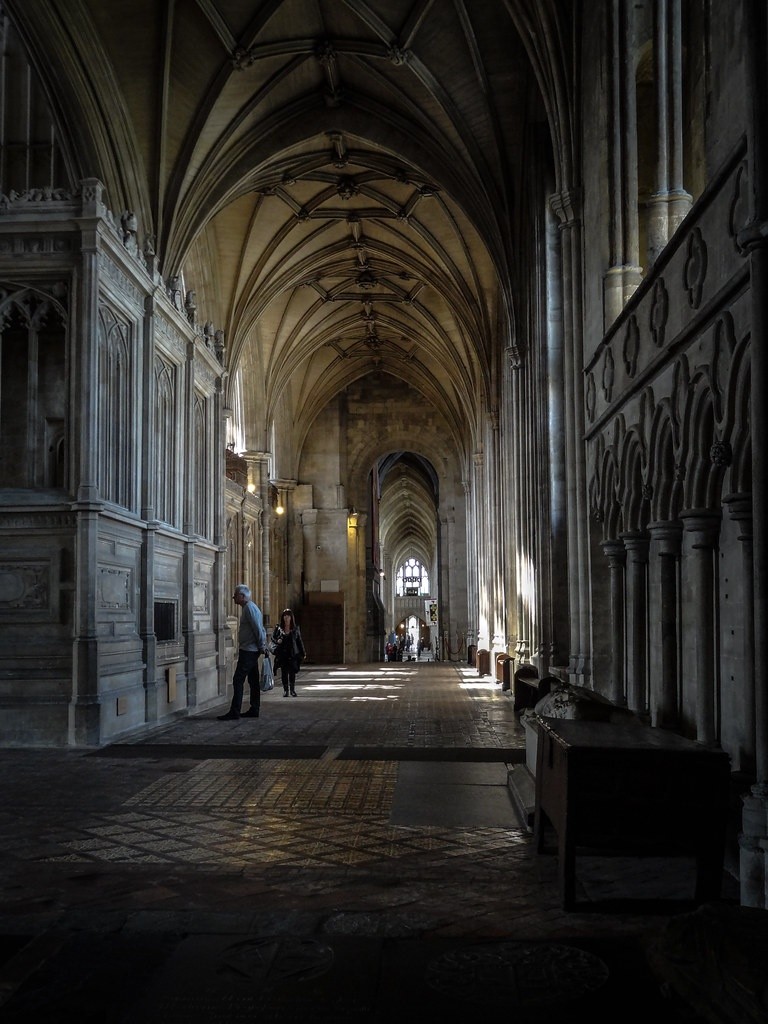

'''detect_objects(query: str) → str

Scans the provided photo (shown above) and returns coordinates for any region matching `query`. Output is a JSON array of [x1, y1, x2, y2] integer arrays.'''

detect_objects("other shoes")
[[240, 707, 259, 718], [290, 692, 297, 697], [216, 708, 240, 719], [283, 689, 288, 697]]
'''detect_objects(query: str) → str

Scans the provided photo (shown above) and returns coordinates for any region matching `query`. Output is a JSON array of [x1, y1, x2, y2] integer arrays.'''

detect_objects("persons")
[[216, 585, 269, 721], [396, 633, 432, 662], [385, 643, 403, 662], [271, 609, 306, 697]]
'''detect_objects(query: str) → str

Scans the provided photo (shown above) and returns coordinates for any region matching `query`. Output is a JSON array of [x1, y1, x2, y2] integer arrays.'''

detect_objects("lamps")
[[351, 506, 358, 515]]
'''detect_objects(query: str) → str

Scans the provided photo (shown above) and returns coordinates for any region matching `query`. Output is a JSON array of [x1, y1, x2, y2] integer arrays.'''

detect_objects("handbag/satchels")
[[259, 650, 274, 691], [268, 624, 281, 654]]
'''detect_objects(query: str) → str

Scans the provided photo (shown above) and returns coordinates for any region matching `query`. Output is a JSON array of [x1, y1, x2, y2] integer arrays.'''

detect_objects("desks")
[[535, 714, 729, 915]]
